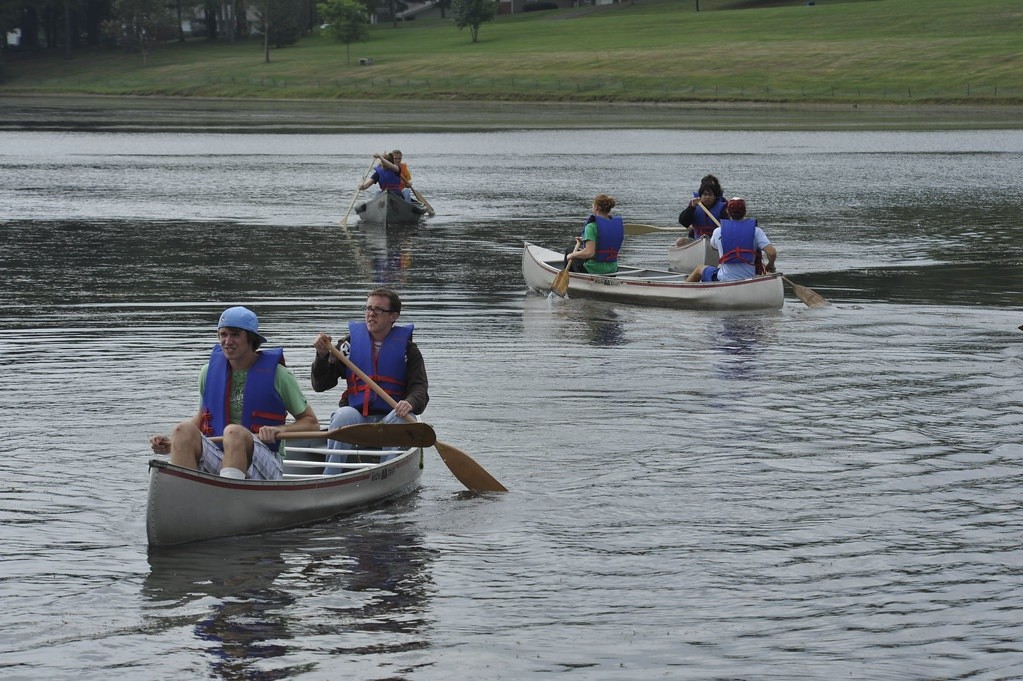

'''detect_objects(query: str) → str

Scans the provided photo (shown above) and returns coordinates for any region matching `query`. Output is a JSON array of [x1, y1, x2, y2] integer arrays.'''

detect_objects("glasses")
[[363, 306, 397, 315]]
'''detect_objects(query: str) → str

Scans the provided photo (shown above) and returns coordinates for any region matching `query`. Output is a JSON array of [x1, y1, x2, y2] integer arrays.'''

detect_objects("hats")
[[218, 307, 268, 344], [727, 200, 746, 216]]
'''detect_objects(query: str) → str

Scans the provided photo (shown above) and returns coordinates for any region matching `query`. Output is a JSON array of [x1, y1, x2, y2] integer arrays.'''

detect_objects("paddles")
[[627, 220, 693, 234], [764, 266, 831, 308], [340, 157, 377, 224], [323, 337, 508, 492], [159, 421, 436, 449], [397, 168, 435, 215], [551, 236, 585, 300], [696, 198, 721, 229]]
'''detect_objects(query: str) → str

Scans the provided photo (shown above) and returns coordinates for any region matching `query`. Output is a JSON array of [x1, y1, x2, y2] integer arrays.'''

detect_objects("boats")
[[521, 240, 786, 313], [145, 411, 425, 548], [356, 183, 426, 229]]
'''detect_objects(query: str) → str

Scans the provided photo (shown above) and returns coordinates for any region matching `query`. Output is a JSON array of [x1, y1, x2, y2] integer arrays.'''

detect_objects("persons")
[[564, 194, 624, 277], [150, 306, 320, 480], [685, 197, 777, 282], [676, 181, 730, 247], [392, 150, 412, 201], [358, 153, 405, 199], [688, 175, 728, 238], [311, 288, 429, 474]]
